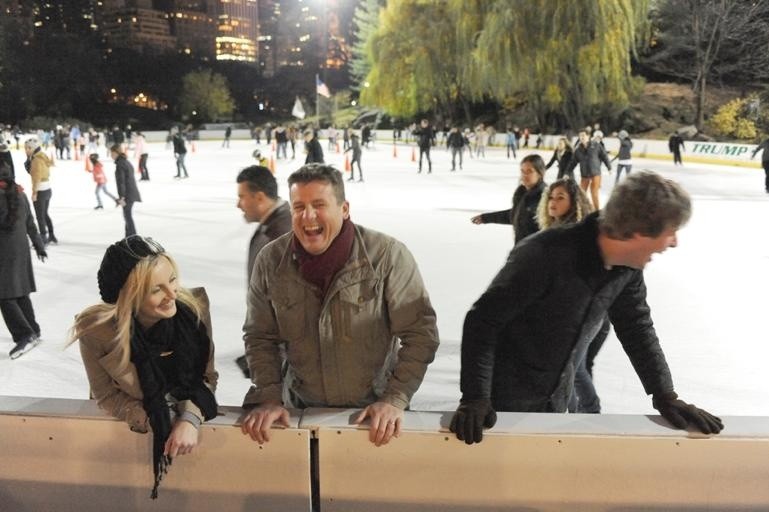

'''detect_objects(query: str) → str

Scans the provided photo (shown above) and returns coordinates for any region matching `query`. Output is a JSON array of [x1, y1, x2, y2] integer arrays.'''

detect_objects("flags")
[[317, 79, 331, 98], [291, 99, 306, 119]]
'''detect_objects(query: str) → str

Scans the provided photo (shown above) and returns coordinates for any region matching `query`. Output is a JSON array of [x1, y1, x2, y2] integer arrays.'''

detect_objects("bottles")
[[98, 236, 165, 304], [25, 139, 39, 149]]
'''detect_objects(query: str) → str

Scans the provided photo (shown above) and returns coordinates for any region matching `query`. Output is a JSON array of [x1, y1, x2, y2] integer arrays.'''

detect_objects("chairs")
[[8, 335, 37, 355]]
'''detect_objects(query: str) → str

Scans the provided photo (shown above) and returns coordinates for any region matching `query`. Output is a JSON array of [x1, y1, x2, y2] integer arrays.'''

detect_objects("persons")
[[535, 178, 597, 232], [470, 154, 547, 245], [448, 169, 724, 446], [526, 123, 633, 211], [408, 121, 530, 174], [750, 139, 768, 194], [110, 145, 143, 237], [63, 234, 219, 460], [668, 131, 686, 166], [87, 154, 121, 209], [250, 122, 375, 182], [2, 144, 16, 180], [1, 123, 137, 162], [172, 129, 189, 178], [235, 165, 292, 378], [23, 138, 58, 242], [1, 161, 47, 357], [133, 131, 150, 180], [240, 162, 441, 447], [222, 125, 232, 148]]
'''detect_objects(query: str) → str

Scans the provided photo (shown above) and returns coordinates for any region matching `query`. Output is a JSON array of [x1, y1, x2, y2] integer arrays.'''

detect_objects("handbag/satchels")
[[177, 411, 201, 430]]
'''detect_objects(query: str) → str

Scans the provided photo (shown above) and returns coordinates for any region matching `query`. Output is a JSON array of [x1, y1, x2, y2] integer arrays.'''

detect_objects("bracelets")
[[177, 411, 201, 430]]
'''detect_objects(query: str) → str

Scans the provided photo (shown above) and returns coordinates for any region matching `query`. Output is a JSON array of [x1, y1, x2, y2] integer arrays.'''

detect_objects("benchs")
[[291, 99, 306, 119], [317, 79, 331, 98]]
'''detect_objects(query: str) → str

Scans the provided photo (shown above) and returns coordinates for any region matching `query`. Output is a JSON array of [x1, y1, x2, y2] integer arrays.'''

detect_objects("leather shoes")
[[653, 391, 723, 434], [449, 399, 496, 444]]
